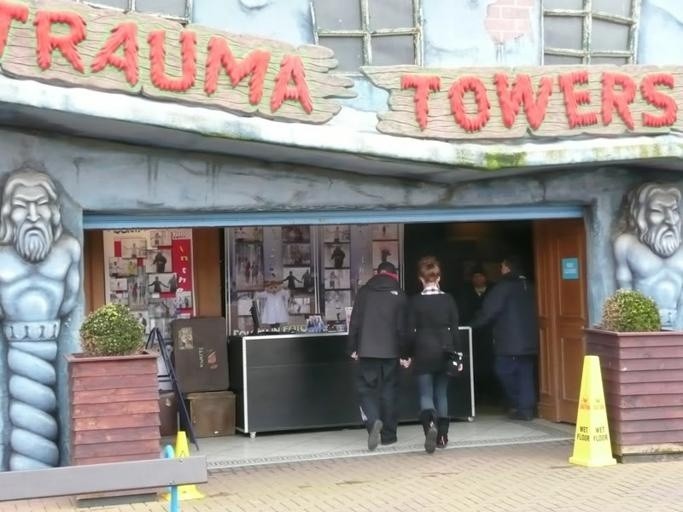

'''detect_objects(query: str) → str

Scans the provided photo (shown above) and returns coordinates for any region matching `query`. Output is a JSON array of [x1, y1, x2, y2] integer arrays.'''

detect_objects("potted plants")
[[64, 302, 161, 500], [582, 286, 683, 463]]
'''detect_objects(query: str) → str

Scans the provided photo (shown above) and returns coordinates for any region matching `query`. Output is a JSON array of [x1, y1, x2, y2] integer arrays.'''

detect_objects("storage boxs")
[[183, 391, 236, 437]]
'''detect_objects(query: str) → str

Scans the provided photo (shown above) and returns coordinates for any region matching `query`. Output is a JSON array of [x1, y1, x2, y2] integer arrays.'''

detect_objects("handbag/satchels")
[[447, 352, 464, 377]]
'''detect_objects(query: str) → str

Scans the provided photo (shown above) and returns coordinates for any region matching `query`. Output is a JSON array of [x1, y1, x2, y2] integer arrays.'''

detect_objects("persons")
[[613, 178, 683, 332], [465, 269, 498, 406], [0, 168, 81, 325], [110, 230, 190, 339], [406, 255, 464, 453], [462, 244, 539, 420], [233, 228, 347, 331], [348, 261, 409, 452]]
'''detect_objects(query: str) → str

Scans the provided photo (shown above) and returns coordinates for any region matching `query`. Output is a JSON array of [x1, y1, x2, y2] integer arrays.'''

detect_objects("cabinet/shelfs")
[[225, 223, 405, 336], [227, 326, 476, 438]]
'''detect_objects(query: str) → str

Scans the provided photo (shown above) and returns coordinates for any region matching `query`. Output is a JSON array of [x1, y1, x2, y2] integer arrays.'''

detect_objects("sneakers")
[[421, 410, 437, 452], [500, 404, 537, 422], [437, 418, 449, 447], [383, 433, 398, 445], [365, 418, 383, 451]]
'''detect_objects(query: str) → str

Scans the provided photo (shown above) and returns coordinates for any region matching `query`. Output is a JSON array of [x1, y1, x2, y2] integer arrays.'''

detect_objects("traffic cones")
[[569, 356, 618, 467], [160, 431, 204, 501]]
